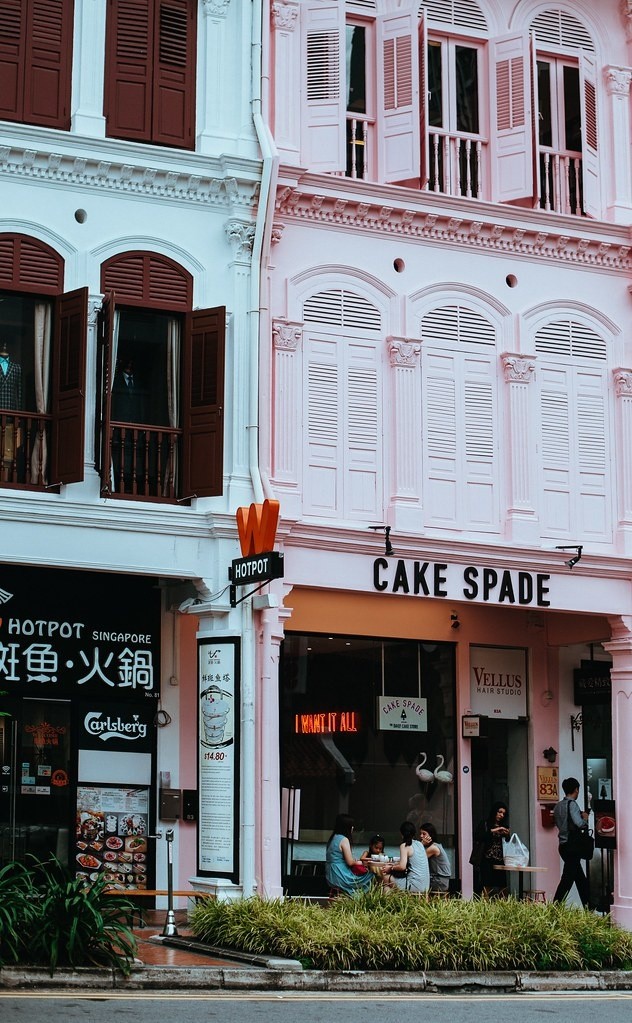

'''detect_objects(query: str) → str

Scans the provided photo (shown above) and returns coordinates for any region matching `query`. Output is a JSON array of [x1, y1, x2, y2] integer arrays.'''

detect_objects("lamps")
[[368, 525, 395, 556], [556, 545, 584, 570], [543, 747, 558, 763]]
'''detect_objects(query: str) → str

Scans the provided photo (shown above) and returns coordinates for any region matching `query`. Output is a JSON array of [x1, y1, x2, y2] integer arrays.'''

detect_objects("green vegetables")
[[133, 838, 144, 844], [84, 854, 89, 860]]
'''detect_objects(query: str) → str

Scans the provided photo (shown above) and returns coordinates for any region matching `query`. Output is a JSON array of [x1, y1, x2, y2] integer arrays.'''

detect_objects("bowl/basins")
[[371, 854, 379, 859], [393, 857, 400, 861]]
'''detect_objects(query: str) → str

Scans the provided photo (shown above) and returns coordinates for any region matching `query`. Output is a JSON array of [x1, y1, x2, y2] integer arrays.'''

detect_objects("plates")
[[595, 817, 616, 837], [76, 811, 146, 890]]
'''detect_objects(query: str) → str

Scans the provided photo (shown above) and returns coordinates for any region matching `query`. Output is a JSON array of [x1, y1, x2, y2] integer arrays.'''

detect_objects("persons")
[[420, 823, 451, 891], [553, 778, 593, 912], [325, 814, 374, 899], [380, 821, 430, 895], [474, 800, 509, 899], [360, 835, 387, 860]]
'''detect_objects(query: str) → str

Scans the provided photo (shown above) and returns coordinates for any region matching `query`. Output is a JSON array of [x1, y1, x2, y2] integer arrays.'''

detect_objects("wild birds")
[[416, 752, 452, 785]]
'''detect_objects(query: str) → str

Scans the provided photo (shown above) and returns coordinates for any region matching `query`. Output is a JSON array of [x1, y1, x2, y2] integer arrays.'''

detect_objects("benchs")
[[82, 888, 218, 928]]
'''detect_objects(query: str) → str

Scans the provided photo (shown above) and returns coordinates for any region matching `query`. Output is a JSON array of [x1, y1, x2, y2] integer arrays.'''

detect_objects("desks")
[[493, 864, 548, 903], [363, 861, 399, 866]]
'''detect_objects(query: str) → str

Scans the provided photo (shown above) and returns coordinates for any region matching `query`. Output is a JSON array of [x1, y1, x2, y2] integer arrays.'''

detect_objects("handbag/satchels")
[[502, 833, 529, 869], [390, 862, 411, 879], [350, 865, 368, 876], [565, 800, 595, 860]]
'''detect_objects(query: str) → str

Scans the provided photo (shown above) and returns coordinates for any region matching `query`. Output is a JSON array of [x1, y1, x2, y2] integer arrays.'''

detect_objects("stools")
[[330, 887, 342, 899], [430, 891, 448, 901], [524, 889, 546, 903]]
